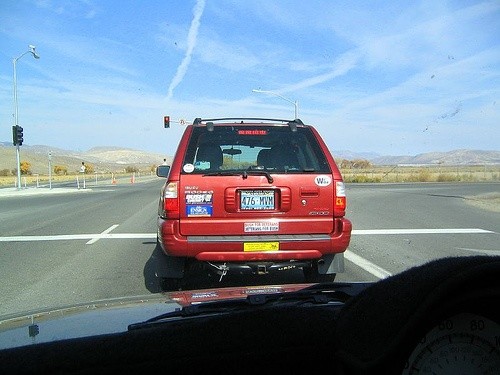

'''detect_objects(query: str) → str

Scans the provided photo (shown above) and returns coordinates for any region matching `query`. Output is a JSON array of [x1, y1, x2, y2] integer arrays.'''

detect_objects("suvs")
[[153, 115, 354, 292]]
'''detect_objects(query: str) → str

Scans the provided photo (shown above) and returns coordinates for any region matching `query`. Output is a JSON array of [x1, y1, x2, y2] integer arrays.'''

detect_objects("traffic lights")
[[12, 125, 24, 145], [164, 116, 170, 128]]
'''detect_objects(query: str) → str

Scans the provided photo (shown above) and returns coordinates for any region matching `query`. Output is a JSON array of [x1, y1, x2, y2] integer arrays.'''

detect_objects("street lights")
[[250, 86, 299, 118], [12, 44, 41, 189]]
